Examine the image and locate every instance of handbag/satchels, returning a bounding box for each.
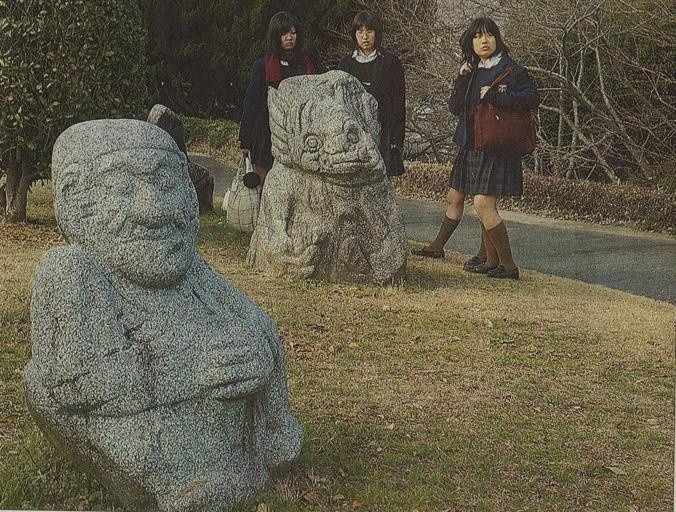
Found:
[224,153,263,233]
[470,101,536,156]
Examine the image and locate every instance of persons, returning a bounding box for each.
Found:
[238,11,312,198]
[337,10,406,179]
[25,117,305,512]
[460,17,537,278]
[410,30,491,272]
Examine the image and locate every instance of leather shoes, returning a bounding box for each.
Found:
[463,254,522,280]
[410,244,445,258]
